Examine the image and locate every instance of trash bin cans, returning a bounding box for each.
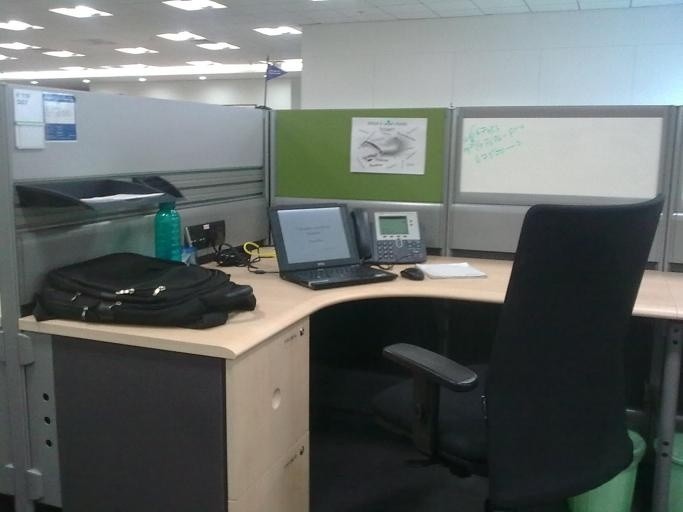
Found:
[566,428,683,512]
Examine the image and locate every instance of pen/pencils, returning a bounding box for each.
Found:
[184,226,193,248]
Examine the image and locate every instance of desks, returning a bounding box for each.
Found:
[18,248,683,512]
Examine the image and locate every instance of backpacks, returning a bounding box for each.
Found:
[32,253,256,329]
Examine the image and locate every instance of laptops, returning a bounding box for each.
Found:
[267,204,397,290]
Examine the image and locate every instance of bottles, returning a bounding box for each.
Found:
[155,200,181,262]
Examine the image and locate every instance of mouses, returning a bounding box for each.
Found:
[400,268,424,280]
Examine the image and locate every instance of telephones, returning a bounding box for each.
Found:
[350,208,427,264]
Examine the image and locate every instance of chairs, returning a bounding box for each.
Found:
[372,194,666,511]
